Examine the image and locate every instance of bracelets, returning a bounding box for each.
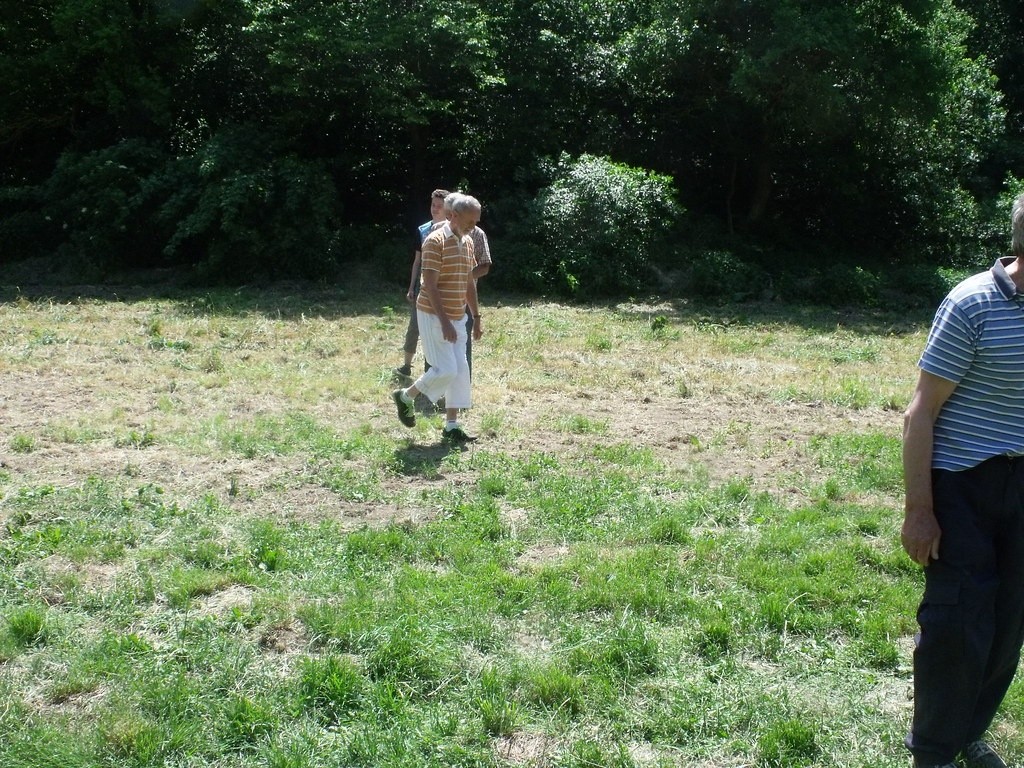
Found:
[472,314,482,319]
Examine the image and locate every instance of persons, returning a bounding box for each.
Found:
[902,189,1024,768]
[392,189,451,377]
[392,196,484,442]
[425,192,492,381]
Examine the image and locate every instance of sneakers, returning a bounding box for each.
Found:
[442,427,479,444]
[392,388,416,427]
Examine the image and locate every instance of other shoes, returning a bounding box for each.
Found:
[395,364,413,376]
[963,737,1008,768]
[910,753,960,768]
